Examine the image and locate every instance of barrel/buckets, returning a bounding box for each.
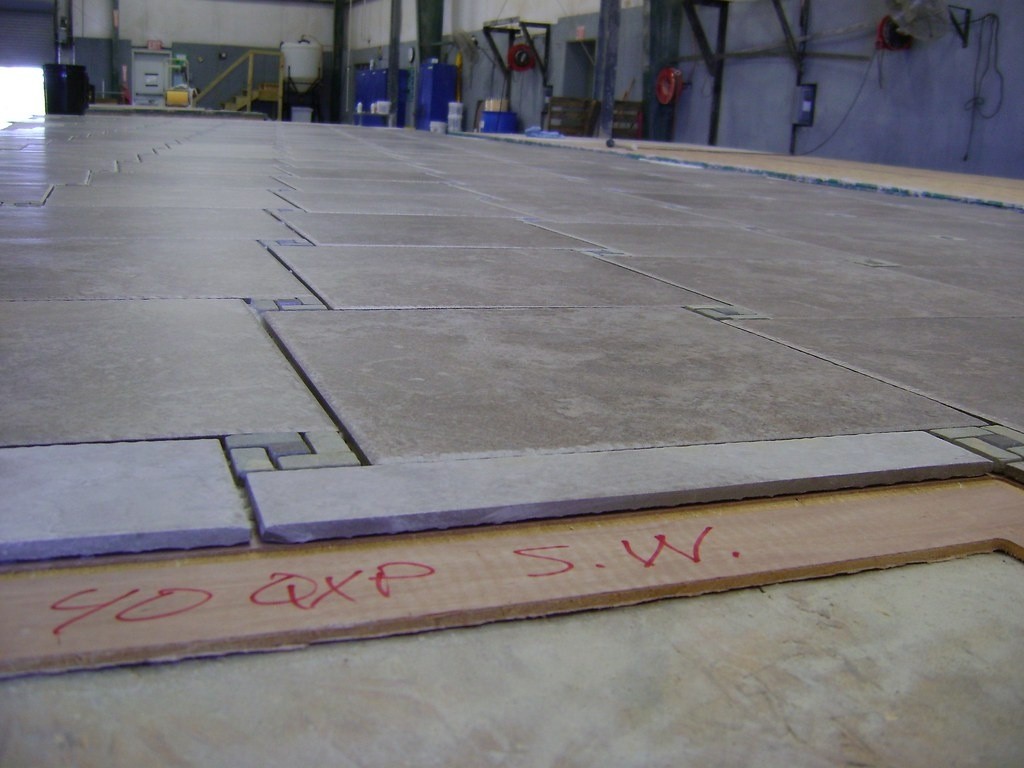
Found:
[429,121,448,134]
[448,103,464,133]
[478,110,519,135]
[376,101,392,114]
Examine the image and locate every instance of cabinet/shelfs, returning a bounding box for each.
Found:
[130,47,172,106]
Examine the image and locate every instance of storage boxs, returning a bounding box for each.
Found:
[485,98,510,111]
[480,110,517,134]
[43,63,89,116]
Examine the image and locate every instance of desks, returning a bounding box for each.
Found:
[415,63,457,132]
[356,69,409,127]
[353,111,392,127]
